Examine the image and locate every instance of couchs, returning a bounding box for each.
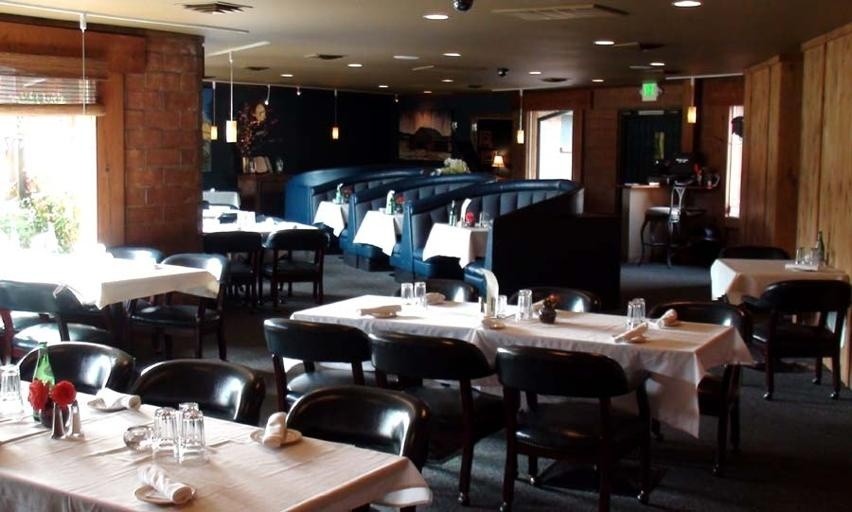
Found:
[285,166,586,299]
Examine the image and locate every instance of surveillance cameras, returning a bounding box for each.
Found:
[451,0,474,14]
[496,67,509,78]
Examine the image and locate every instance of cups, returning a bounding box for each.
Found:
[2,361,25,417]
[794,245,822,266]
[396,280,655,330]
[122,401,207,459]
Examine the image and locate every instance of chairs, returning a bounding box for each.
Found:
[329,280,755,512]
[641,180,722,268]
[0,203,329,512]
[712,245,851,402]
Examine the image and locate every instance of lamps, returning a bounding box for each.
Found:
[491,155,506,175]
[224,47,241,145]
[330,86,341,141]
[515,88,526,145]
[685,75,701,127]
[207,78,223,143]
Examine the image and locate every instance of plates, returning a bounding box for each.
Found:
[135,483,196,503]
[249,429,302,448]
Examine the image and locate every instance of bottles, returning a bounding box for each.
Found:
[334,186,494,227]
[813,230,825,263]
[50,400,87,440]
[33,339,53,424]
